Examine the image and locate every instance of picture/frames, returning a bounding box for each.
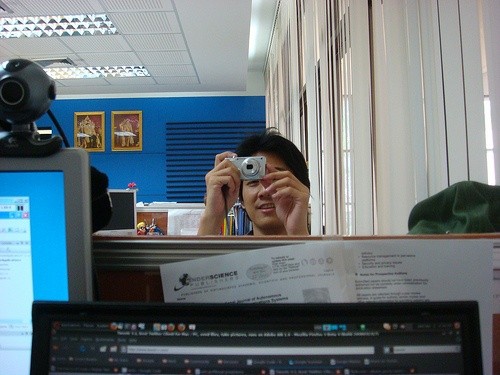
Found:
[110,111,142,152]
[73,111,105,152]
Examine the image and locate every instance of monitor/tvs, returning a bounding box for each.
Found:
[31,299,484,375]
[94,190,136,237]
[0,148,95,375]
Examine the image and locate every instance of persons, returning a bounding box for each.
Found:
[197,127,310,237]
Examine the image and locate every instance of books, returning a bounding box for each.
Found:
[218,206,250,236]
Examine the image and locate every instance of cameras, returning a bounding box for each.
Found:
[223,155,268,180]
